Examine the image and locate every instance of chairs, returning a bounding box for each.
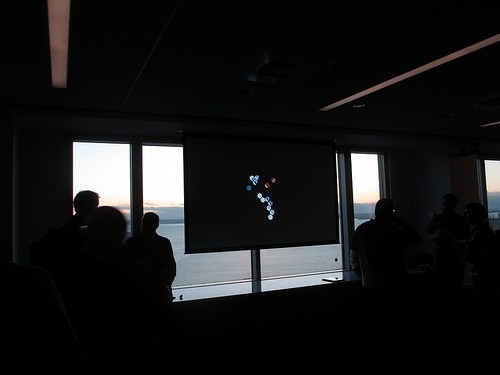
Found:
[0,261,95,375]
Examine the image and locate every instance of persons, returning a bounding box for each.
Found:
[427,194,500,326]
[63,190,176,305]
[350,198,421,299]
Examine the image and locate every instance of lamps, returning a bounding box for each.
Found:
[47,0,71,89]
[318,32,500,112]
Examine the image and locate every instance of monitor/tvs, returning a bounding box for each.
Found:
[182,131,339,254]
